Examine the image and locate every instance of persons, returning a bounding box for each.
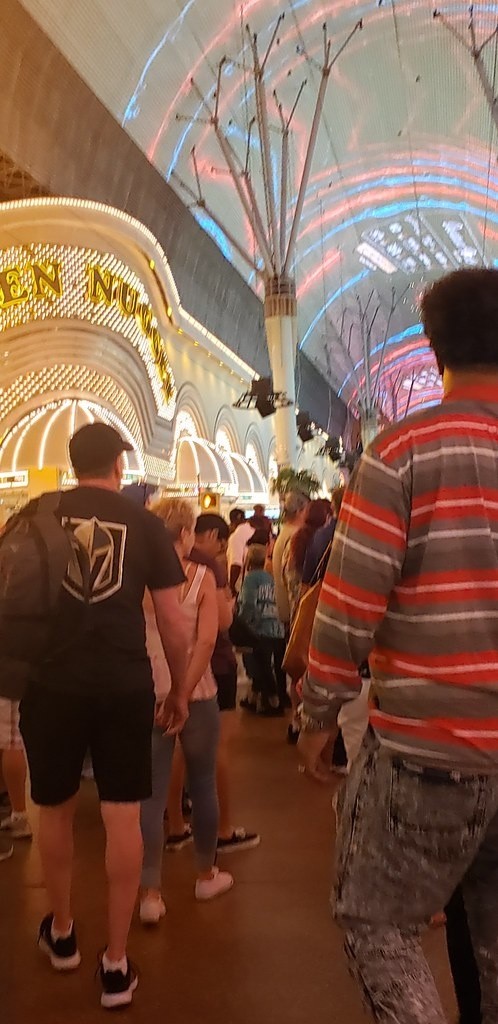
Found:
[0,485,373,925]
[0,422,189,1004]
[297,271,498,1023]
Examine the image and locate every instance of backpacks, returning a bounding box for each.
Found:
[0,489,91,700]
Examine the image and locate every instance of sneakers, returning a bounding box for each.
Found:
[94,944,139,1008]
[37,911,81,970]
[166,822,193,851]
[216,825,260,854]
[140,895,165,925]
[195,865,234,900]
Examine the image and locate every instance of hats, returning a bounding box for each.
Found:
[122,482,160,507]
[70,423,134,471]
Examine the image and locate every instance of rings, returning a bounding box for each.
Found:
[298,765,306,773]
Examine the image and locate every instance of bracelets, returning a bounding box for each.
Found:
[297,703,323,731]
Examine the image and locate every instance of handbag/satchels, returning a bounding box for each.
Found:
[228,617,259,648]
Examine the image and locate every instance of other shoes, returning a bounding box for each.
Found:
[0,835,13,861]
[259,704,284,717]
[0,817,32,840]
[240,698,256,711]
[280,690,292,708]
